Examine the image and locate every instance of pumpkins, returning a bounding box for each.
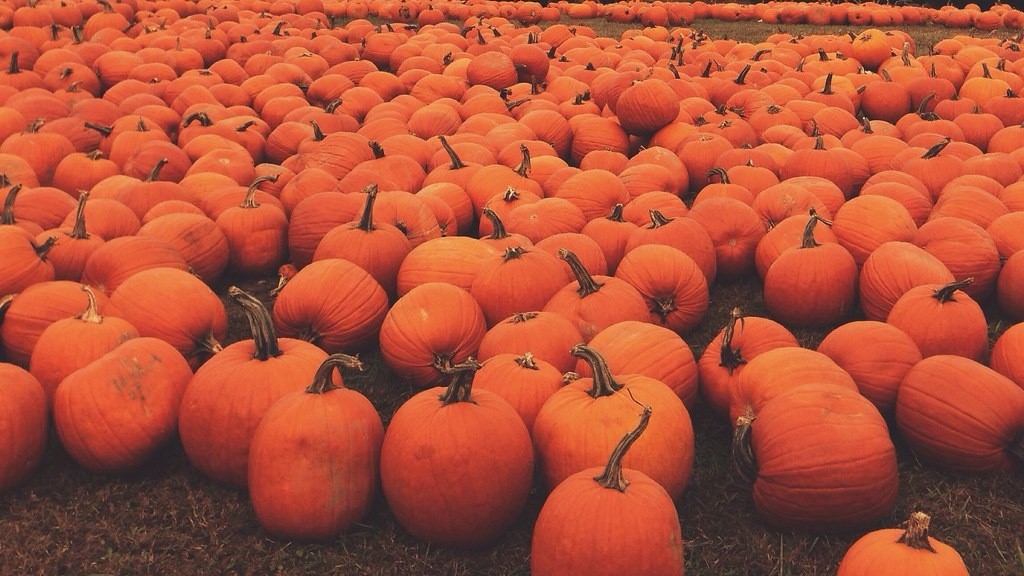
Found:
[0,0,1024,576]
